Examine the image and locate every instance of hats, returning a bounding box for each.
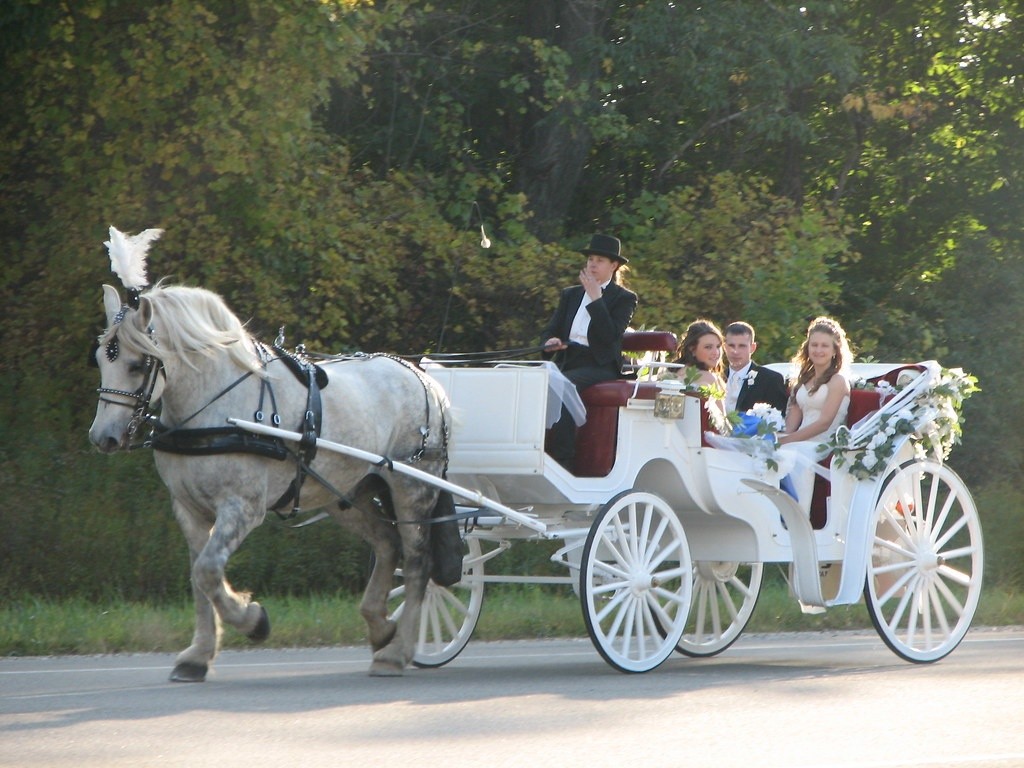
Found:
[580,234,629,265]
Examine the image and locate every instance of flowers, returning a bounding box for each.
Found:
[835,359,982,481]
[748,404,785,431]
[623,322,679,384]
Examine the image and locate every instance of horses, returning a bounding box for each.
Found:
[88,226,465,682]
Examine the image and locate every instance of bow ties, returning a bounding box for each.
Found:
[601,287,604,294]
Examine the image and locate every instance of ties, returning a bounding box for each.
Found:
[729,373,739,410]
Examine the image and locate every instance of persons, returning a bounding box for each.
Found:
[539,234,639,469]
[666,316,856,522]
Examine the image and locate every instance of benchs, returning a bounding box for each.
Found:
[544,381,663,476]
[620,332,731,448]
[782,388,904,481]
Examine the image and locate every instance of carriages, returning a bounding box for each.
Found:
[88,227,987,680]
